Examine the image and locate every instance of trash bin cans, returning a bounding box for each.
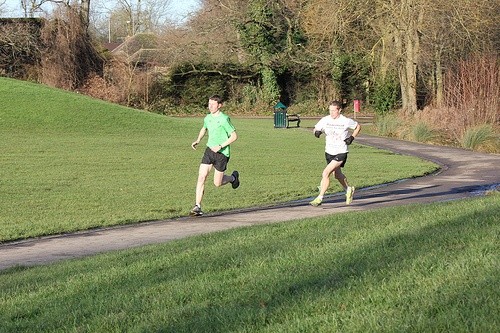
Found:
[273,102,287,129]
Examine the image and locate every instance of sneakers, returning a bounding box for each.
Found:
[230,170,239,188]
[345,186,355,205]
[309,197,323,207]
[190,204,203,215]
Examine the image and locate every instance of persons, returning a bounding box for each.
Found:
[309,101,361,207]
[190,95,240,216]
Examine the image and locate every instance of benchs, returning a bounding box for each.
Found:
[272,111,301,128]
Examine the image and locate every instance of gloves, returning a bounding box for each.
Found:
[315,130,323,138]
[344,135,354,145]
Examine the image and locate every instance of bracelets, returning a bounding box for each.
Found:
[219,144,222,149]
